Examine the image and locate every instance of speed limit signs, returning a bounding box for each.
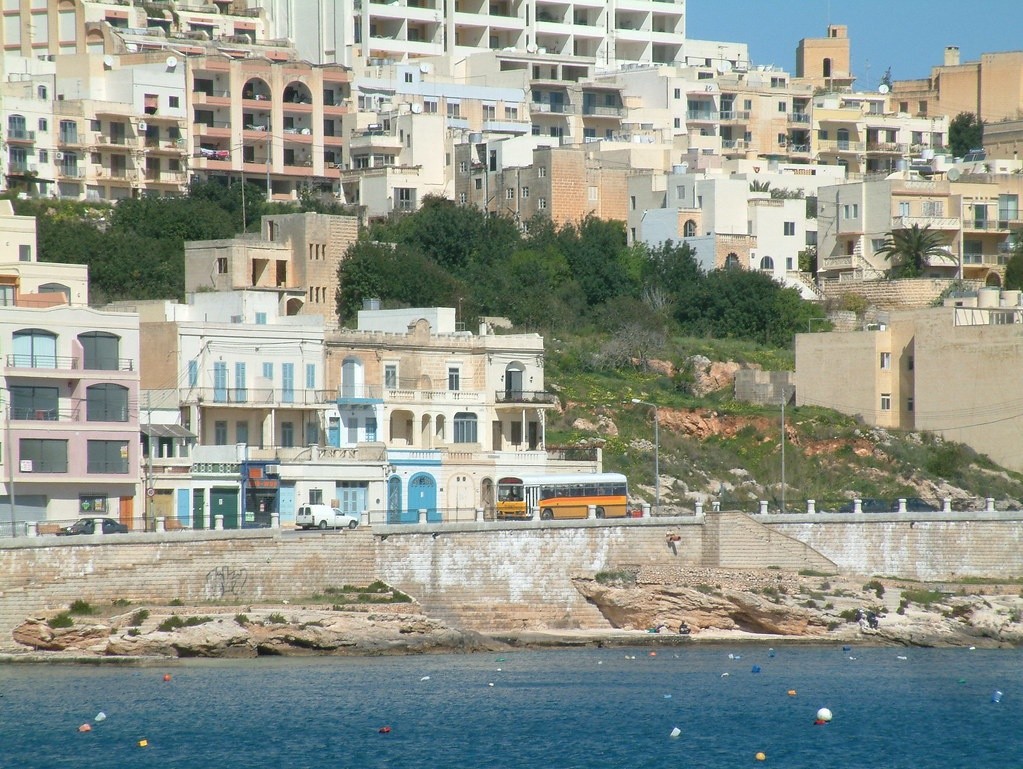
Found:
[146,488,155,496]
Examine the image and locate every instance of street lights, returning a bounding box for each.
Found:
[632,398,659,515]
[781,388,787,512]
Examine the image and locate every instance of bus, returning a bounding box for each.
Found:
[486,472,628,520]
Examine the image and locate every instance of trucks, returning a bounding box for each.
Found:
[296,504,359,529]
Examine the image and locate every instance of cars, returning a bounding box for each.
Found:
[838,497,889,514]
[57,517,127,534]
[891,497,939,513]
